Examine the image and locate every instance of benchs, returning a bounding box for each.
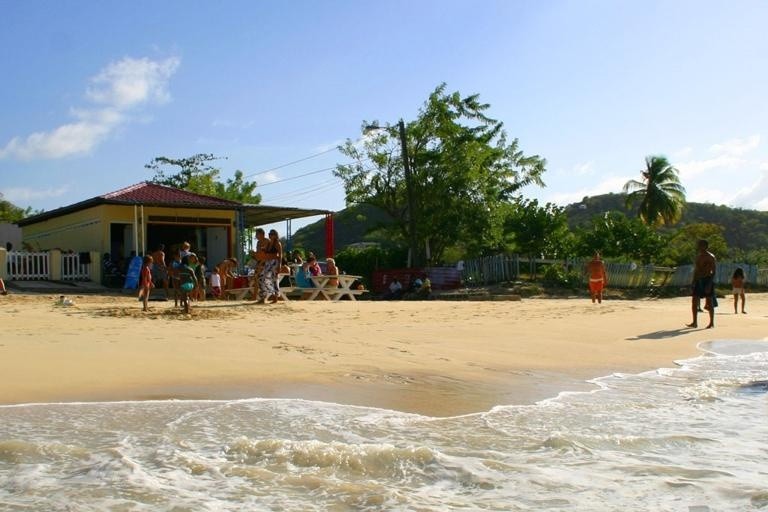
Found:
[224,285,371,300]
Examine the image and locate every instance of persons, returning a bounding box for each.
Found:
[102,228,347,314]
[687,239,716,328]
[403,275,423,299]
[731,268,747,314]
[584,252,607,304]
[416,273,432,300]
[382,277,403,300]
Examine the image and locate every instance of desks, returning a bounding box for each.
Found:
[306,274,363,300]
[237,272,290,300]
